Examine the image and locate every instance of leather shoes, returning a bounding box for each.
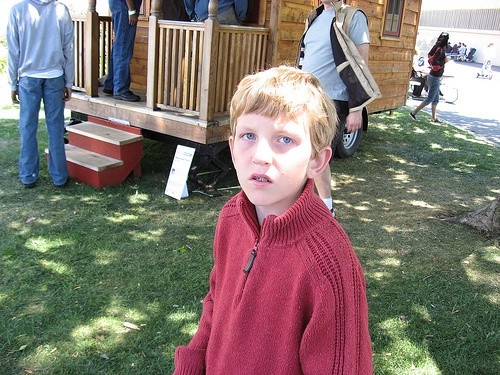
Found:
[103,88,114,94]
[24,180,36,188]
[113,89,141,101]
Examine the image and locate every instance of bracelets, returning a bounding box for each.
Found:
[128,10,137,15]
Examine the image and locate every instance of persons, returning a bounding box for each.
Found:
[172,63,374,375]
[6,0,76,189]
[407,32,452,123]
[446,41,476,63]
[183,0,248,28]
[102,0,142,103]
[292,0,371,218]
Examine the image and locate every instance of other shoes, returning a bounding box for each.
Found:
[431,119,443,124]
[409,112,416,120]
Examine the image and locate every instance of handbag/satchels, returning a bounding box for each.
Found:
[427,47,443,71]
